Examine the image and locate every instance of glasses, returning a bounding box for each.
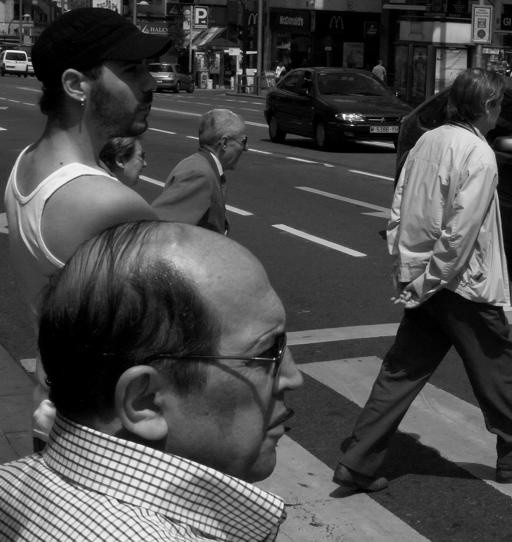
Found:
[230,134,248,147]
[138,331,292,372]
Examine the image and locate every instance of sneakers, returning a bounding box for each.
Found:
[331,462,392,494]
[495,467,511,484]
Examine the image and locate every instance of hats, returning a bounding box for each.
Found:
[30,7,178,83]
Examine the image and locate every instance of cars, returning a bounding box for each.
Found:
[0,49,34,79]
[141,62,194,93]
[264,66,419,152]
[394,75,512,246]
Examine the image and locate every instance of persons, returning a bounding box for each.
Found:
[2,6,164,454]
[0,217,306,542]
[150,105,250,240]
[331,68,510,492]
[99,134,152,188]
[371,59,387,84]
[275,61,286,82]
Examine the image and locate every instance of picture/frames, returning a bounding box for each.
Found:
[471,4,492,45]
